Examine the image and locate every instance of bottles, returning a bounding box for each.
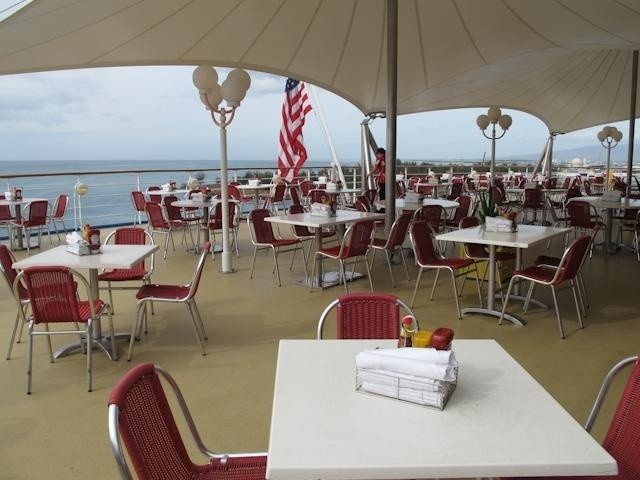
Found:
[169,180,172,190]
[397,316,412,349]
[11,186,16,195]
[322,196,329,205]
[330,200,338,218]
[82,224,91,241]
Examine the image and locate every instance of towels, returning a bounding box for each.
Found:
[354,346,461,409]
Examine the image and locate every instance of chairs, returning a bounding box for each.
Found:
[106,362,269,480]
[496,353,640,480]
[130,172,639,339]
[1,194,211,395]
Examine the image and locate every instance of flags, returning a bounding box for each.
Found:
[273,77,313,184]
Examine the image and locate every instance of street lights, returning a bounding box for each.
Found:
[476,105,512,214]
[192,63,252,274]
[596,126,623,193]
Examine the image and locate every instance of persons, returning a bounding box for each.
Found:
[366,147,385,214]
